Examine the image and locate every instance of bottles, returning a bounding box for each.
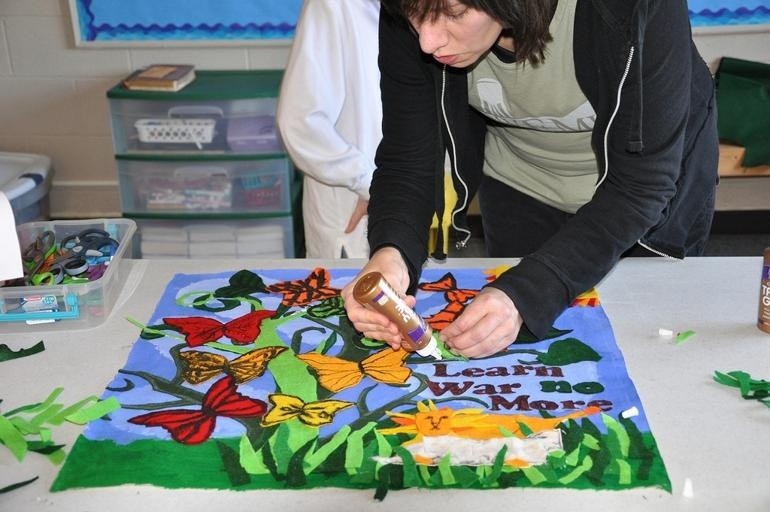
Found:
[757,246,770,332]
[353,273,442,361]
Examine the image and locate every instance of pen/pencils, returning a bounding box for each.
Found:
[147,189,232,210]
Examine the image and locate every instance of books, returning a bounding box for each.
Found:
[138,223,283,260]
[122,63,198,92]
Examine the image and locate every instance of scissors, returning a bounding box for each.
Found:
[3,228,119,306]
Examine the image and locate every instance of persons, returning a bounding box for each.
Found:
[340,0,720,359]
[276,1,383,260]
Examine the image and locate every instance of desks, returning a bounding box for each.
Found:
[1,255,770,512]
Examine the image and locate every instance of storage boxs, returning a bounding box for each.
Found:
[103,72,290,156]
[0,152,53,228]
[111,153,301,213]
[122,212,301,260]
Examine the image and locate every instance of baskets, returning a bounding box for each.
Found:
[134,118,216,144]
